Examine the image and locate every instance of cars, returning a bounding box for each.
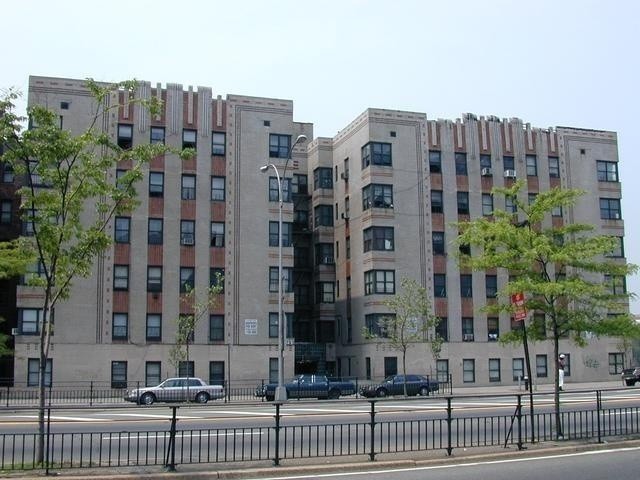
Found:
[125,377,226,403]
[256,374,439,401]
[622,367,640,386]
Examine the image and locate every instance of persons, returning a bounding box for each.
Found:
[557,354,567,390]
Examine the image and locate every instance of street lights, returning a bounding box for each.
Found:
[259,134,308,402]
